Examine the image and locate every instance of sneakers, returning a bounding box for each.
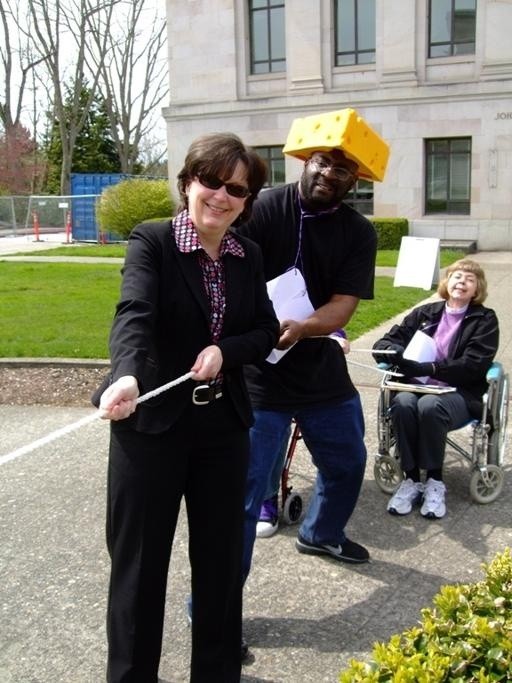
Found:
[256,493,280,536]
[295,531,368,564]
[421,479,446,519]
[387,479,424,515]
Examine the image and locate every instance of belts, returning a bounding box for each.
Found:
[192,385,224,406]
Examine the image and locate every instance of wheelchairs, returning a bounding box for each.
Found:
[372,359,510,503]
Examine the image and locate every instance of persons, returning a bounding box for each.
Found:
[371,259,499,518]
[91,131,281,683]
[256,425,292,537]
[235,149,378,564]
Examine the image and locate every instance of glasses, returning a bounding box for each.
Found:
[196,174,253,198]
[312,158,357,182]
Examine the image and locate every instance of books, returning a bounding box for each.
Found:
[383,380,456,395]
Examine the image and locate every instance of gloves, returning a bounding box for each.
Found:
[385,343,405,364]
[395,358,435,380]
